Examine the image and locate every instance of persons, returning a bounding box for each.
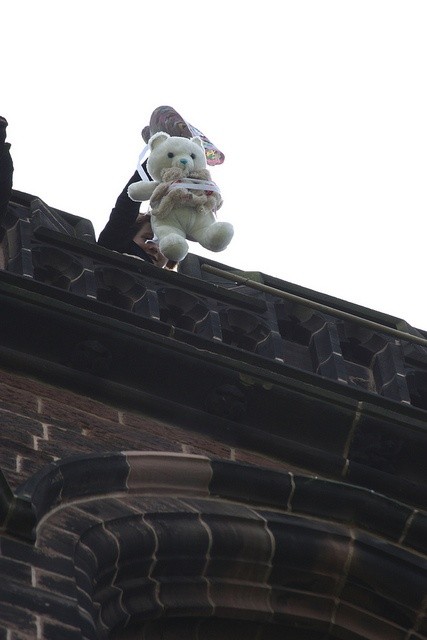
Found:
[97,157,176,269]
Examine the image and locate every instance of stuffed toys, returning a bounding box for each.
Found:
[127,131,234,263]
